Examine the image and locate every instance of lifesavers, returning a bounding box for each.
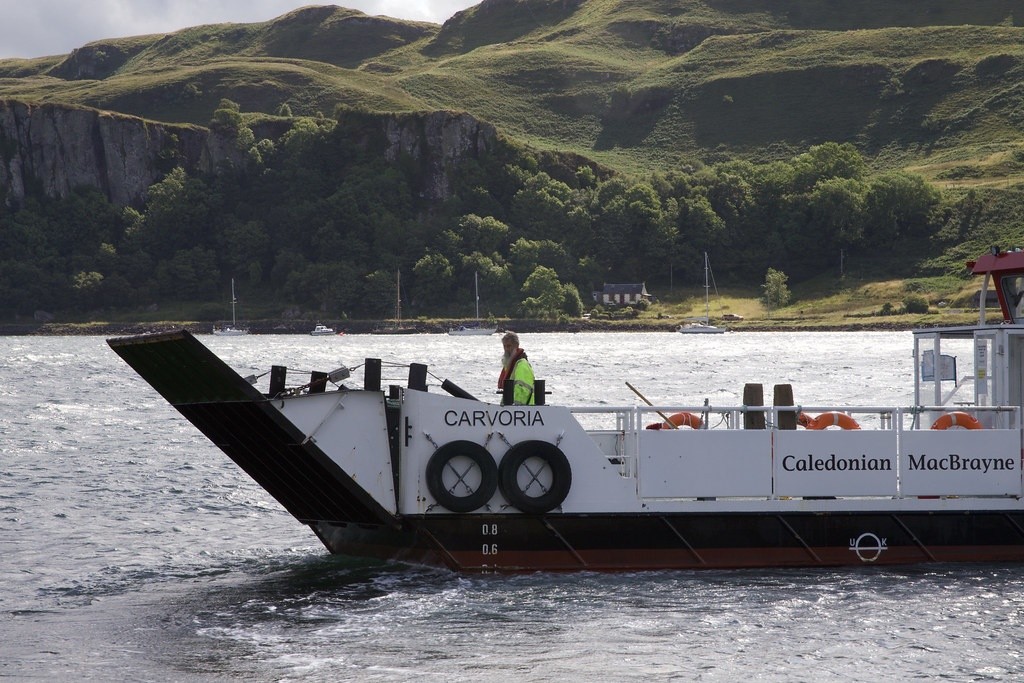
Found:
[425,440,498,512]
[796,412,827,430]
[807,412,861,431]
[498,439,572,513]
[931,412,984,429]
[662,413,705,430]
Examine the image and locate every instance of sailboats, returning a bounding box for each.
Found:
[675,252,726,333]
[449,269,497,335]
[371,266,425,335]
[212,278,251,335]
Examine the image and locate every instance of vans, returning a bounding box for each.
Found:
[582,314,591,320]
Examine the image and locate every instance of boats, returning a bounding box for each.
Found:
[311,325,337,335]
[105,249,1024,576]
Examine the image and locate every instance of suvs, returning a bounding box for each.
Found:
[722,314,743,321]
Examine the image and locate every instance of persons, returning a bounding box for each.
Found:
[498,332,535,406]
